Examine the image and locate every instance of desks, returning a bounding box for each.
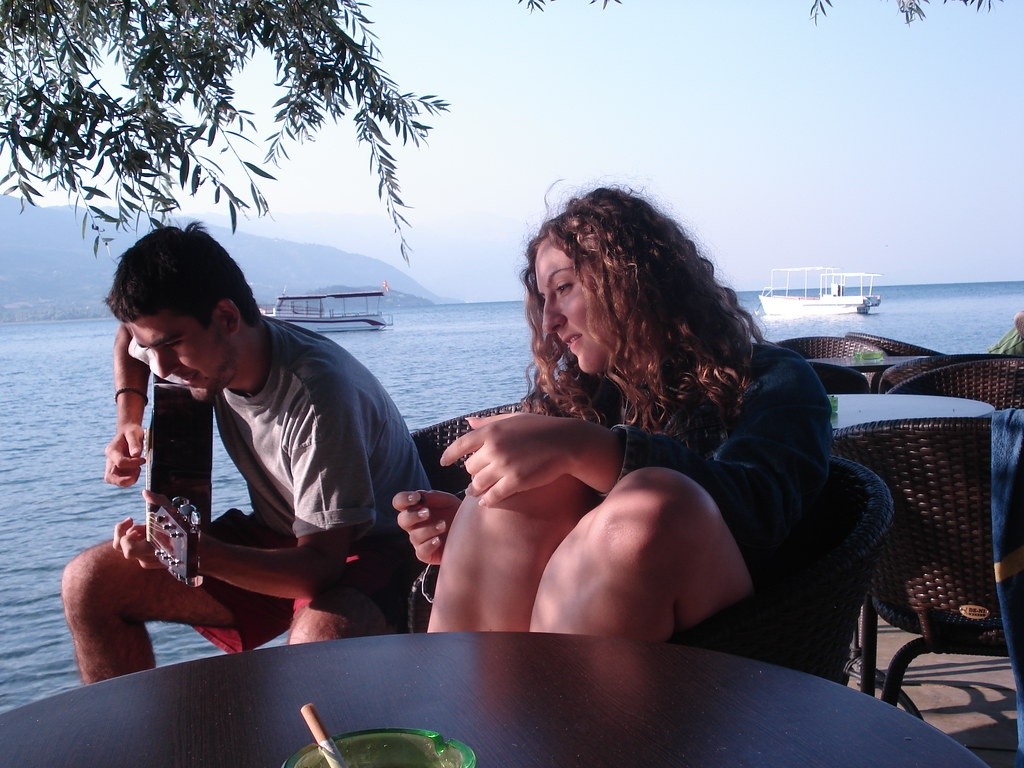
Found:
[819,385,996,696]
[0,620,992,768]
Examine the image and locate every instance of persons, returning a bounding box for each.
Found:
[988,311,1024,356]
[61,188,835,683]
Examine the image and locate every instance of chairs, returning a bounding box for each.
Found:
[386,329,1024,711]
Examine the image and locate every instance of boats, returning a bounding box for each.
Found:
[261,288,394,335]
[758,266,882,317]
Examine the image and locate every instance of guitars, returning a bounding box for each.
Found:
[144,373,214,588]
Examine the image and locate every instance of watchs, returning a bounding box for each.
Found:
[115,387,148,406]
